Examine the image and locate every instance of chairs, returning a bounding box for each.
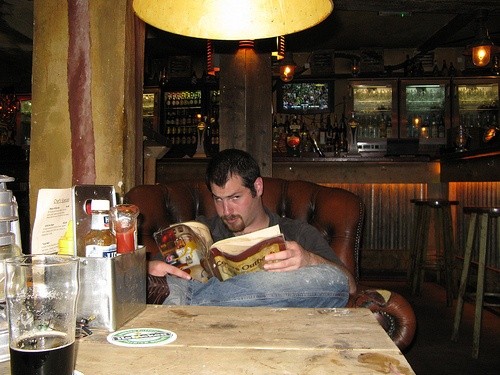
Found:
[122,178,414,352]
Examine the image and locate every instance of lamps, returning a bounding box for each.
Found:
[463,11,500,67]
[239,40,255,49]
[279,52,296,82]
[133,0,334,41]
[278,35,285,56]
[207,39,215,76]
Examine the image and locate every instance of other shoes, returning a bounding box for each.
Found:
[146,272,170,305]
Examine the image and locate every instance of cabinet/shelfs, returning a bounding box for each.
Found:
[143,81,220,157]
[348,76,500,150]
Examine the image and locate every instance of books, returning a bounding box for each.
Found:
[154,222,287,282]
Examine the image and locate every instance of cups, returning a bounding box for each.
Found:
[4,254,80,375]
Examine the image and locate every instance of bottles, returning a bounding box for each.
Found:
[432,60,439,77]
[110,204,139,254]
[269,111,359,158]
[455,113,500,154]
[82,199,117,258]
[143,60,219,158]
[449,62,456,77]
[56,220,75,256]
[403,54,423,77]
[357,113,393,139]
[442,60,448,76]
[405,111,445,140]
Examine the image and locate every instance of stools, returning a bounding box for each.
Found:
[453,207,500,360]
[410,198,458,314]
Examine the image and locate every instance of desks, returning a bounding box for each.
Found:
[0,304,415,375]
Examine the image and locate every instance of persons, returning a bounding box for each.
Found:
[147,149,355,308]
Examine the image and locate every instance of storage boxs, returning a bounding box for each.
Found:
[44,246,146,332]
